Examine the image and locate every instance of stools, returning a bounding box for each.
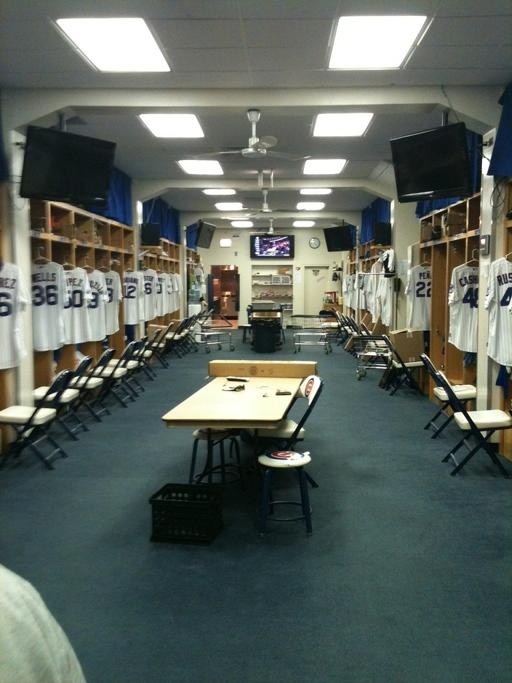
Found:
[258,451,313,539]
[189,427,241,489]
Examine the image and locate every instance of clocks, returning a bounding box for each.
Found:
[309,237,320,248]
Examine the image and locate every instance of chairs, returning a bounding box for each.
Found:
[435,371,511,479]
[0,369,68,470]
[197,308,214,325]
[335,311,348,346]
[381,335,424,396]
[240,375,325,488]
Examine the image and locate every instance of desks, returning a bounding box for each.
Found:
[249,303,285,351]
[161,376,303,522]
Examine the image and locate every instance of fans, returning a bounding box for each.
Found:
[190,108,312,234]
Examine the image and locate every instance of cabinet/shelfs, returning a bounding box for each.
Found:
[343,241,392,336]
[252,265,293,328]
[419,184,512,463]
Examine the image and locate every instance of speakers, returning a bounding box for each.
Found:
[141,223,161,246]
[374,222,391,245]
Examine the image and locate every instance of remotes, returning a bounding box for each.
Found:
[227,376,250,382]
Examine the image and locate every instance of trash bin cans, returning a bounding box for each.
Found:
[256,323,275,353]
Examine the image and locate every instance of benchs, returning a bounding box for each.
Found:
[237,324,303,343]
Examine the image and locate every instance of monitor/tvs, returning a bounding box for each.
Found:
[19,125,117,208]
[323,224,356,252]
[389,123,484,204]
[186,221,216,249]
[250,234,294,259]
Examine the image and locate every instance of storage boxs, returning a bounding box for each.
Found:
[390,328,424,363]
